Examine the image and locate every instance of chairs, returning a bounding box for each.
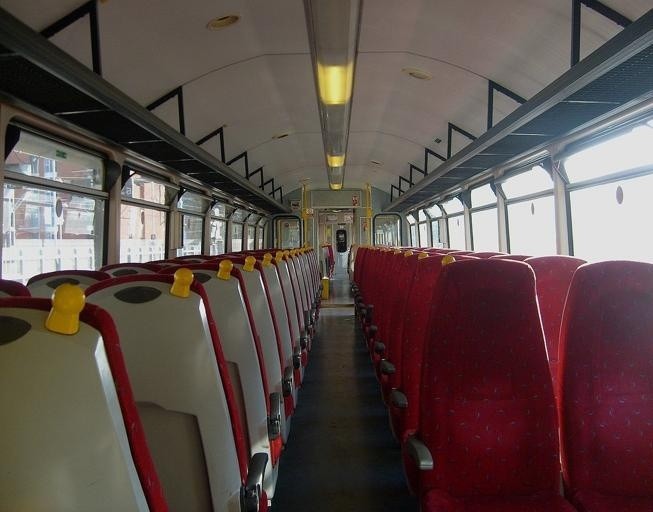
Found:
[351,246,653,512]
[2,246,322,511]
[321,243,335,279]
